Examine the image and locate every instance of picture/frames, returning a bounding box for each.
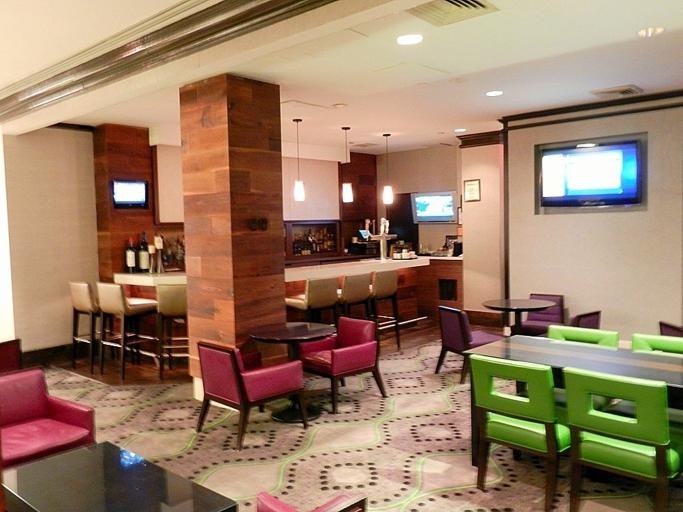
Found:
[462,179,481,202]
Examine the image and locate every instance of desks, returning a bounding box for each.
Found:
[0,440,239,512]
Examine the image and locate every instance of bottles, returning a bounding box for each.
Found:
[124,237,137,273]
[291,227,334,256]
[441,243,447,250]
[136,230,151,273]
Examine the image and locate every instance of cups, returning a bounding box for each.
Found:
[391,249,415,260]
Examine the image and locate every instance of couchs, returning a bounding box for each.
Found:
[0,370,96,472]
[256,487,369,512]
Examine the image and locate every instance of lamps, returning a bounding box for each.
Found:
[292,118,304,200]
[381,133,394,204]
[341,126,354,203]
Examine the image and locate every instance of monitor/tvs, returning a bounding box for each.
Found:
[112,180,148,208]
[410,191,457,224]
[534,131,647,215]
